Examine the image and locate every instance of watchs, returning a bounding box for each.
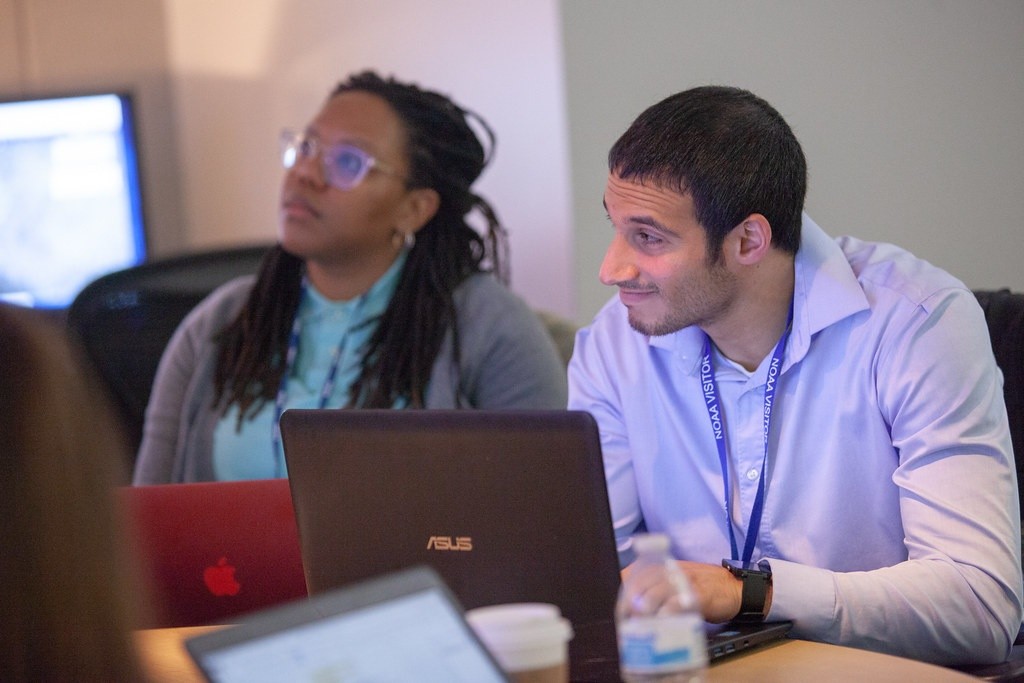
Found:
[722,559,771,626]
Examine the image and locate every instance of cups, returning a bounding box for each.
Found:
[464,605,573,682]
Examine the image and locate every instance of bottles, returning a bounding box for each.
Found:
[614,531,712,683]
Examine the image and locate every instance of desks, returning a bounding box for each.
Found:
[132,619,983,683]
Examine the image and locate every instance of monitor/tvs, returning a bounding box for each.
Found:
[0,94,148,313]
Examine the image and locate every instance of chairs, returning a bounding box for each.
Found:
[0,235,1024,682]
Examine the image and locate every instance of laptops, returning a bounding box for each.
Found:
[113,408,796,683]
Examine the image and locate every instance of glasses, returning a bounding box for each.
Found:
[282,132,412,190]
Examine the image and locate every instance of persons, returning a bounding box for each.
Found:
[564,86,1024,666]
[126,70,567,487]
